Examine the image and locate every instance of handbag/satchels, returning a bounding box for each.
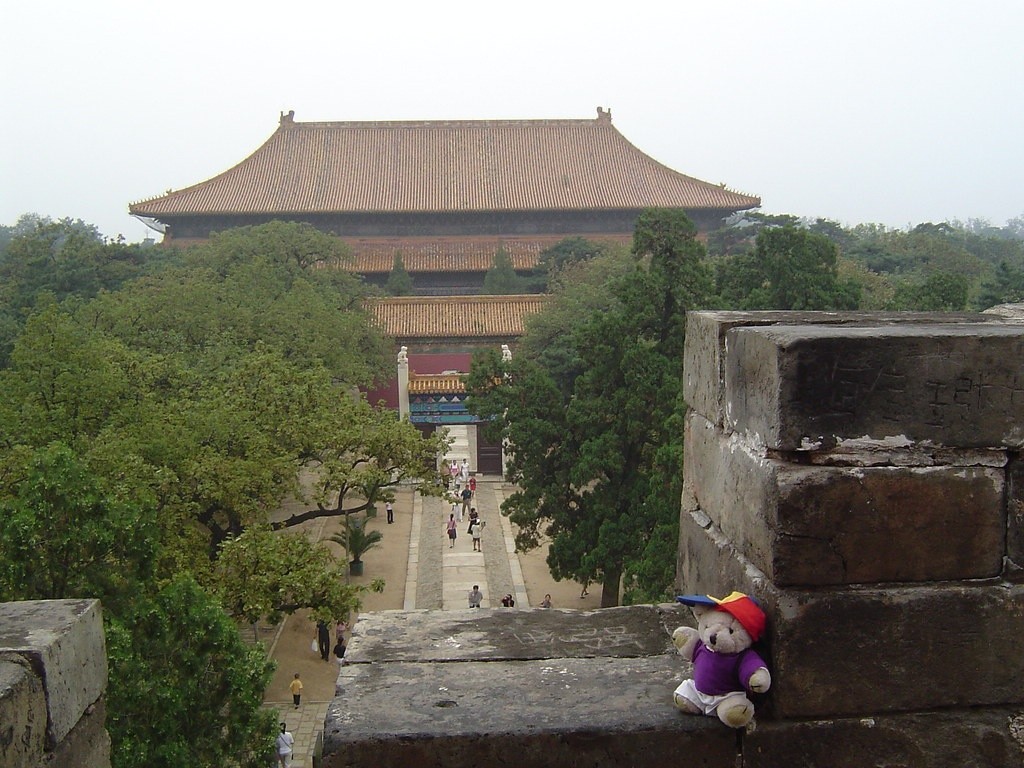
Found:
[312,639,318,652]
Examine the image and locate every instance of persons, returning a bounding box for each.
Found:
[437,456,487,553]
[501,593,514,607]
[538,594,553,608]
[384,493,398,525]
[274,722,295,768]
[310,598,349,667]
[288,673,305,711]
[445,513,457,547]
[467,584,484,608]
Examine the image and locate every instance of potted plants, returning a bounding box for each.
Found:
[331,515,385,576]
[343,487,398,518]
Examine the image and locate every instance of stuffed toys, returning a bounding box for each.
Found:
[669,589,771,729]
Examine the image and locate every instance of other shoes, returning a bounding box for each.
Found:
[473,548,477,551]
[450,545,454,548]
[478,550,481,552]
[295,706,298,709]
[461,520,463,522]
[388,521,394,524]
